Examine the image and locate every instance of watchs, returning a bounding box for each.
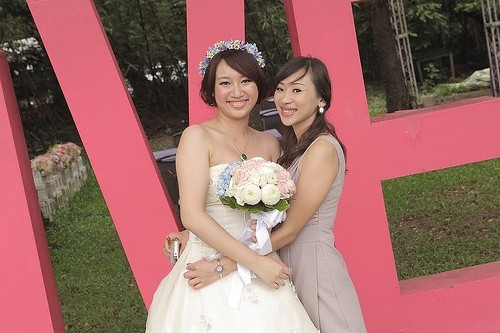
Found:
[215,258,224,279]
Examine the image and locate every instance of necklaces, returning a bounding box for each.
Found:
[216,116,253,161]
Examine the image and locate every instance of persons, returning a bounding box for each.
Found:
[182,56,369,333]
[144,38,322,333]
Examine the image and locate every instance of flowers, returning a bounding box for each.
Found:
[225,157,297,206]
[31,142,82,173]
